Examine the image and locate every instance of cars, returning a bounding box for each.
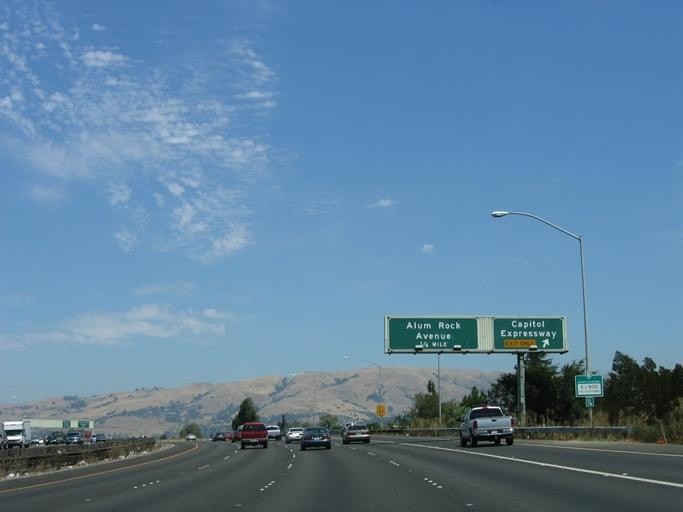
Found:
[210,422,332,450]
[186,434,196,442]
[30,431,112,445]
[341,422,369,444]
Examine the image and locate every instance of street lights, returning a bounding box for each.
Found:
[343,356,382,434]
[492,211,594,422]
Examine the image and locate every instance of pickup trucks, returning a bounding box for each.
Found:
[456,405,514,447]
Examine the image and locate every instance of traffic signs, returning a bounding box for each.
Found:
[384,315,569,355]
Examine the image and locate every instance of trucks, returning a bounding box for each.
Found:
[0,421,31,449]
